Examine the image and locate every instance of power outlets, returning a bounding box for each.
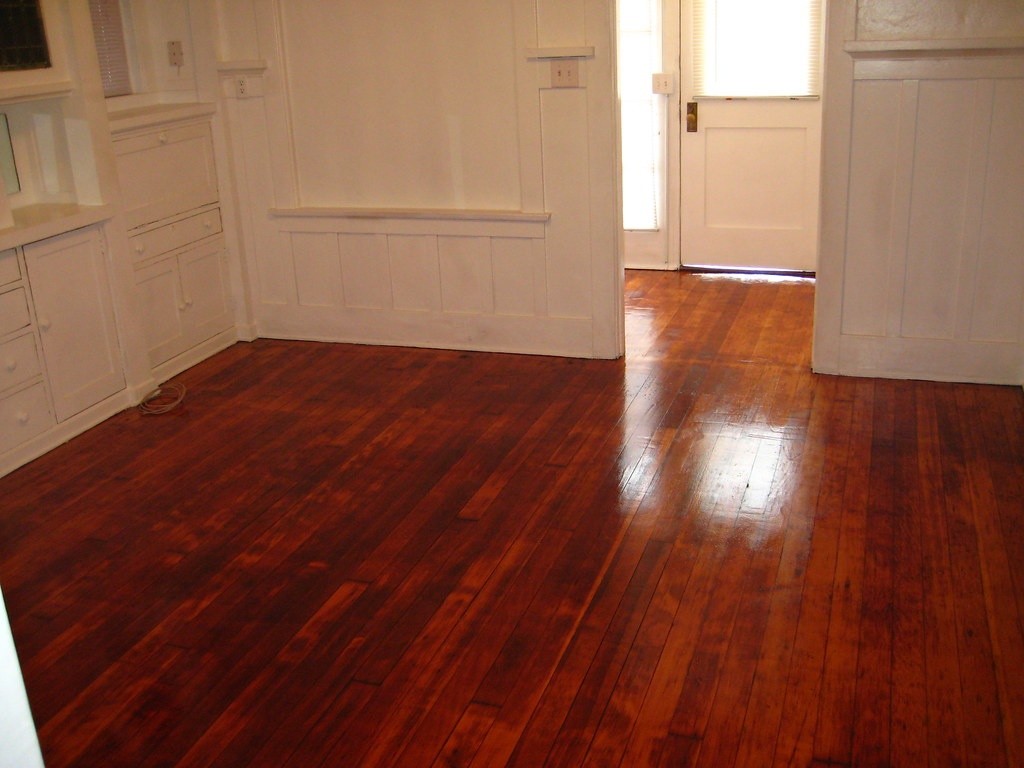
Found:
[237,76,249,98]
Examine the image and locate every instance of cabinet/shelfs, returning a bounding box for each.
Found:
[24,220,127,425]
[102,117,236,372]
[1,248,54,456]
